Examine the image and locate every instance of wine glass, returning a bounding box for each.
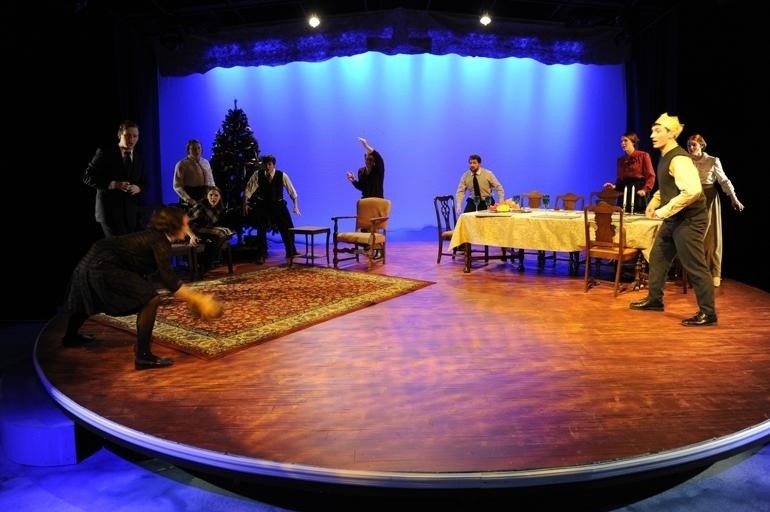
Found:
[542,194,550,216]
[485,196,492,212]
[474,196,480,214]
[513,192,520,206]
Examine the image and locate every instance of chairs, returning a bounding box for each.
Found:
[331,197,391,270]
[554,193,585,211]
[520,192,547,208]
[178,186,232,284]
[589,185,624,205]
[434,195,465,263]
[583,201,641,297]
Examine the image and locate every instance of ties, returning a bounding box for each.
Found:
[267,173,272,182]
[473,174,482,202]
[124,151,131,178]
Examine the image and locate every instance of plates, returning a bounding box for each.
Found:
[491,208,532,213]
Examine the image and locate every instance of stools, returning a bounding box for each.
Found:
[288,227,330,266]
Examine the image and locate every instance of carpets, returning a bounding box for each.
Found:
[87,262,437,362]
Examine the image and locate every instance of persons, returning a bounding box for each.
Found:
[61,202,221,370]
[83,119,152,237]
[686,132,744,290]
[628,111,718,327]
[601,132,656,216]
[187,186,234,275]
[346,138,385,260]
[243,154,301,265]
[454,154,505,215]
[172,138,216,207]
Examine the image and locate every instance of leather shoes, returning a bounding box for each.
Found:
[713,275,720,287]
[134,357,174,371]
[630,296,664,309]
[681,311,718,325]
[62,334,95,346]
[373,250,383,259]
